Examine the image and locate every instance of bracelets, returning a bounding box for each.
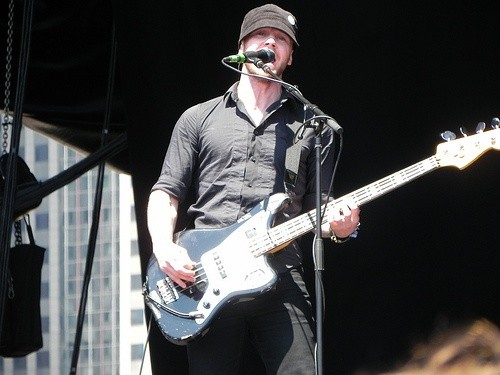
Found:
[329,226,360,244]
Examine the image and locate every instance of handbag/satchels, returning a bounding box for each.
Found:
[0,244,47,357]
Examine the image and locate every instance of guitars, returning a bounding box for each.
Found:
[143,117,500,347]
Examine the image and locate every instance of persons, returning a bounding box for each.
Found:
[147,3,360,375]
[394,320,500,375]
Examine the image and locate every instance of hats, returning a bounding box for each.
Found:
[238,4,301,48]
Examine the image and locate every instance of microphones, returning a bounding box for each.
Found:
[224,48,275,63]
